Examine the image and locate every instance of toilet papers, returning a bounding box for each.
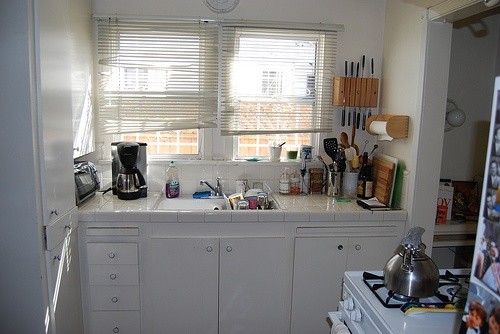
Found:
[369,120,394,142]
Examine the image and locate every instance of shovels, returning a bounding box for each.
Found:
[324,138,338,172]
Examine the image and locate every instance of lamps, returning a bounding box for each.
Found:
[442,97,466,133]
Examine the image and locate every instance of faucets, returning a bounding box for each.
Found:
[200,176,223,199]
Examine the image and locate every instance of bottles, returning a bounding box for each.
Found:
[363,165,373,199]
[356,152,368,198]
[279,168,290,194]
[166,161,179,198]
[290,168,300,194]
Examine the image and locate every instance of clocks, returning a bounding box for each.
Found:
[204,0,240,14]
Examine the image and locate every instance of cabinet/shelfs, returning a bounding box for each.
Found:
[146,235,287,334]
[79,220,146,333]
[289,233,401,334]
[31,0,84,334]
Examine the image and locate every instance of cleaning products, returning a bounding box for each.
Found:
[165,160,179,198]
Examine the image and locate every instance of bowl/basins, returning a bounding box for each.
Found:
[244,188,263,198]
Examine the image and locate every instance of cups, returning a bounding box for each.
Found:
[343,172,359,198]
[287,151,298,159]
[326,170,343,197]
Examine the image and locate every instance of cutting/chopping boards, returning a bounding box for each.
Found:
[371,156,396,206]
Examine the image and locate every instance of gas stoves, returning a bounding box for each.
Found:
[327,268,471,334]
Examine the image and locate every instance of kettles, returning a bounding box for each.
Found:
[383,242,440,298]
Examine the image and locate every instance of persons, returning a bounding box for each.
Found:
[457,115,500,334]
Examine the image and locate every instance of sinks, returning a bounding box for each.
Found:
[155,196,231,220]
